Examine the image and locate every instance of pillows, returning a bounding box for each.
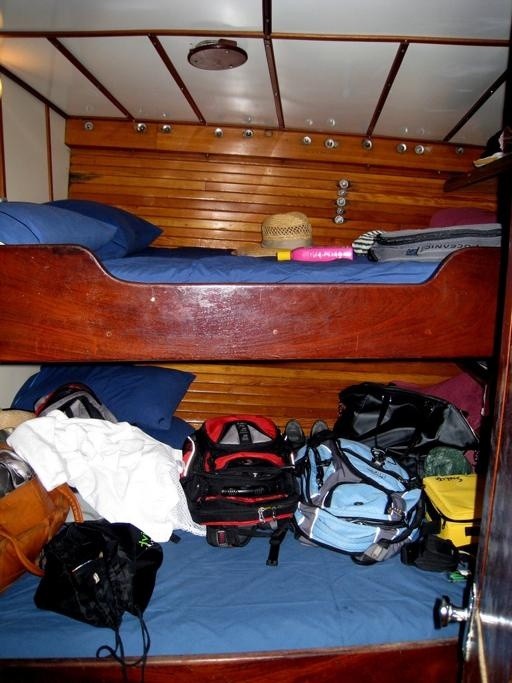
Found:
[2,201,115,258]
[11,365,194,434]
[45,198,161,258]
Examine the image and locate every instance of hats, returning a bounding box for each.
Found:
[232,212,312,260]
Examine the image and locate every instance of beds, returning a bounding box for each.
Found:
[0,248,511,683]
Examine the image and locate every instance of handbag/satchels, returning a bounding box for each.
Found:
[325,370,482,462]
[0,466,82,591]
[395,473,488,572]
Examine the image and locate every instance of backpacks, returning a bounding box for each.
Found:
[284,420,425,566]
[181,415,299,569]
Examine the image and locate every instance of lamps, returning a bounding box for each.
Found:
[187,38,249,72]
